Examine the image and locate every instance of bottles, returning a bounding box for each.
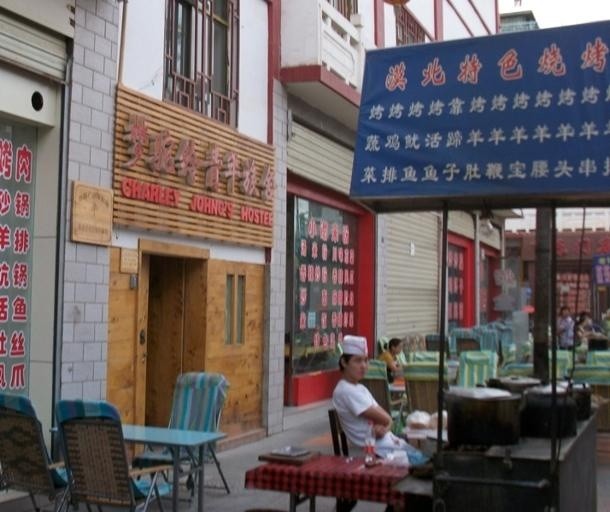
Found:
[363,420,377,466]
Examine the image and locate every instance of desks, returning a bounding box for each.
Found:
[244,452,413,512]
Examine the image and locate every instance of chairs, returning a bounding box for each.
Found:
[1,370,232,512]
[327,408,397,512]
[336,321,610,426]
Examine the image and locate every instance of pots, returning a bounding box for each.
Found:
[441,374,593,447]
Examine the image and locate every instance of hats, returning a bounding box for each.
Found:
[342,335,368,356]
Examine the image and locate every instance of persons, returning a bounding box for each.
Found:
[378,336,405,412]
[556,306,610,353]
[330,333,434,467]
